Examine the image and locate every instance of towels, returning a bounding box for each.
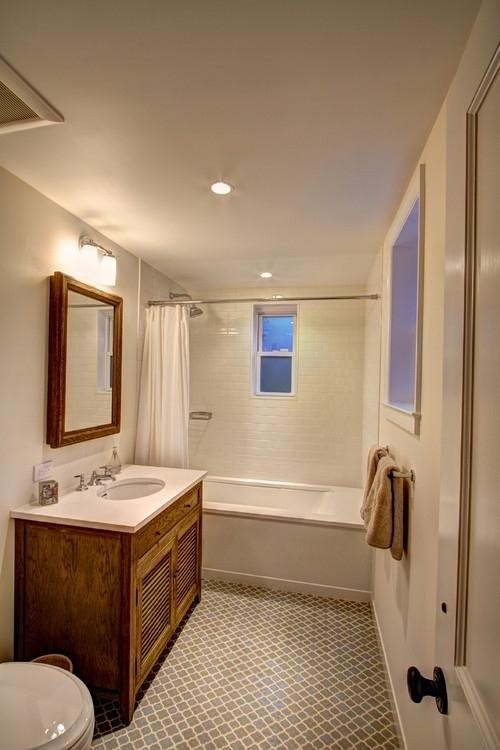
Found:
[360,456,404,560]
[364,446,388,500]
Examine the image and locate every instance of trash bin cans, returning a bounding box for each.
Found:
[29,654,73,673]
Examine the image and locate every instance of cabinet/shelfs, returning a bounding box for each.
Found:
[15,480,203,726]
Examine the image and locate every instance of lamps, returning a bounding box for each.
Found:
[78,234,117,287]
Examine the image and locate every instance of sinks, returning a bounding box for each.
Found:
[95,476,167,502]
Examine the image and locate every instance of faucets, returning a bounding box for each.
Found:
[87,470,107,486]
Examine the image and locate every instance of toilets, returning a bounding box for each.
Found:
[0,659,95,749]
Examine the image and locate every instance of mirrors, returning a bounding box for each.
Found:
[47,271,123,449]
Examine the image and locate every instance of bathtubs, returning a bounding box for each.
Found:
[202,475,375,602]
